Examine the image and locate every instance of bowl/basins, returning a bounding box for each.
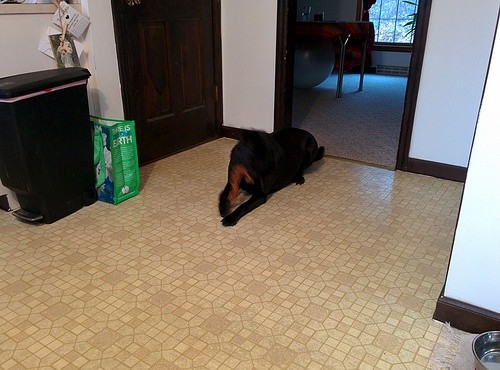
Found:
[472,331,500,370]
[314,14,323,21]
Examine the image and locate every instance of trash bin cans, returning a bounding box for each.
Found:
[0,67,99,226]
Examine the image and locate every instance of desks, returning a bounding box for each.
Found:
[296,20,375,98]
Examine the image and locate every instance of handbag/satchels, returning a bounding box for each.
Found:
[89,115,140,206]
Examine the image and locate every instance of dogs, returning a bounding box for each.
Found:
[218,126,325,227]
